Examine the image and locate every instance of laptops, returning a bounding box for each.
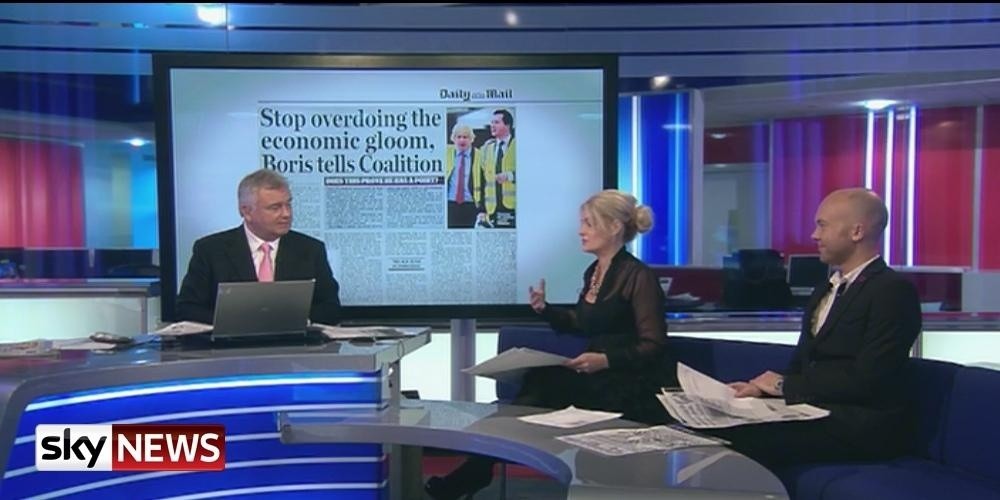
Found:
[177,279,318,348]
[786,253,832,297]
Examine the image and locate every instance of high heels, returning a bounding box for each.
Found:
[426,461,493,499]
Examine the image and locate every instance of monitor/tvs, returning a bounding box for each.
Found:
[150,49,619,323]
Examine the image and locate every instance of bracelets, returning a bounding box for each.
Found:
[774,374,788,394]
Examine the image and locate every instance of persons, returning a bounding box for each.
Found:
[423,187,668,498]
[692,186,922,474]
[446,119,481,228]
[478,109,516,229]
[171,169,345,331]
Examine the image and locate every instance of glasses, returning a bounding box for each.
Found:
[247,198,298,214]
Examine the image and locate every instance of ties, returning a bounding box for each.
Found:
[496,142,505,211]
[457,152,466,205]
[257,243,276,282]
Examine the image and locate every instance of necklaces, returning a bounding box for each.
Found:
[590,260,609,297]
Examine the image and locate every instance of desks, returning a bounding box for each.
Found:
[275,397,791,500]
[0,325,433,500]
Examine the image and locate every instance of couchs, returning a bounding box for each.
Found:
[494,325,1000,500]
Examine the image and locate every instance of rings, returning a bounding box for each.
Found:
[576,367,582,374]
